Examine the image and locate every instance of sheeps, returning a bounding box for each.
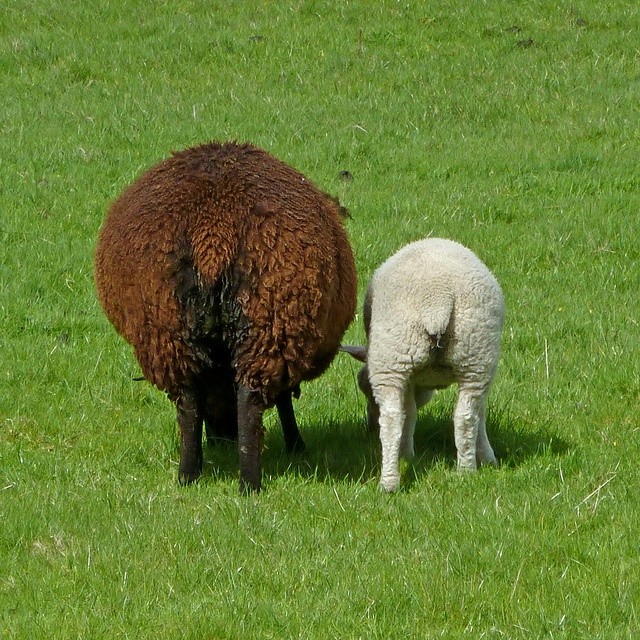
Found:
[337,236,506,494]
[92,137,359,497]
